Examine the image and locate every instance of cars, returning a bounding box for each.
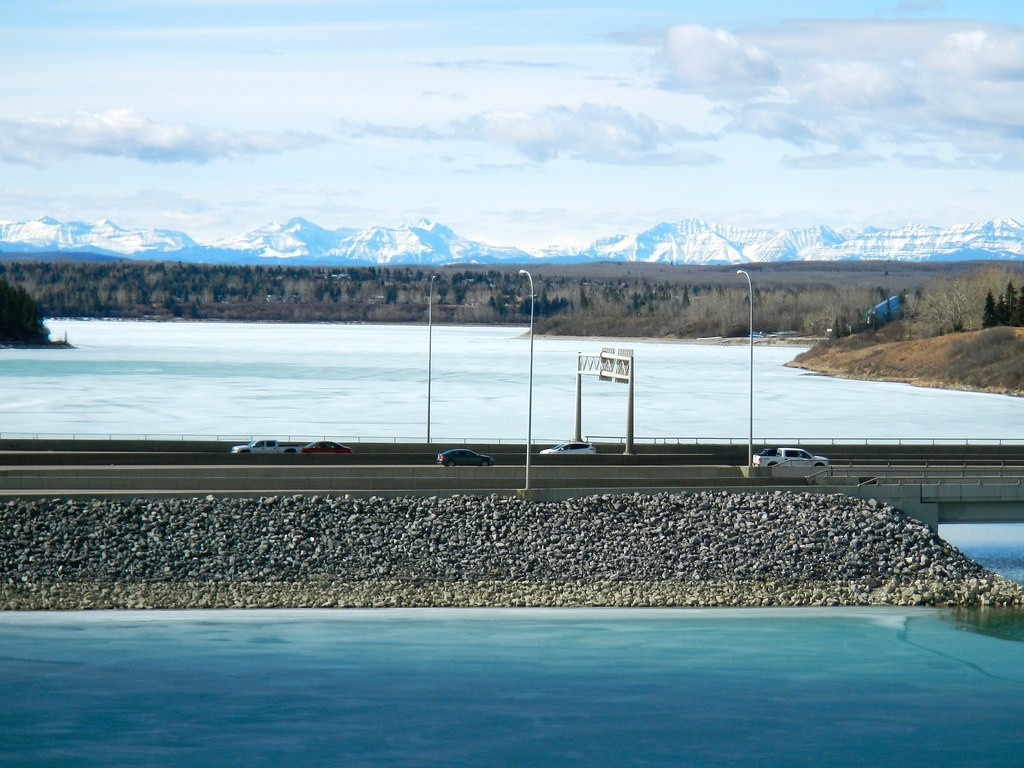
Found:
[302,440,352,453]
[436,448,494,467]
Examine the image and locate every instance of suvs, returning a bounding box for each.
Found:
[540,442,596,454]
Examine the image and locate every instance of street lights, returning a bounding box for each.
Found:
[737,269,753,467]
[427,275,436,444]
[520,269,534,489]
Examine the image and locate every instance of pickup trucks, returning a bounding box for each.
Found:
[231,439,302,453]
[753,447,829,467]
